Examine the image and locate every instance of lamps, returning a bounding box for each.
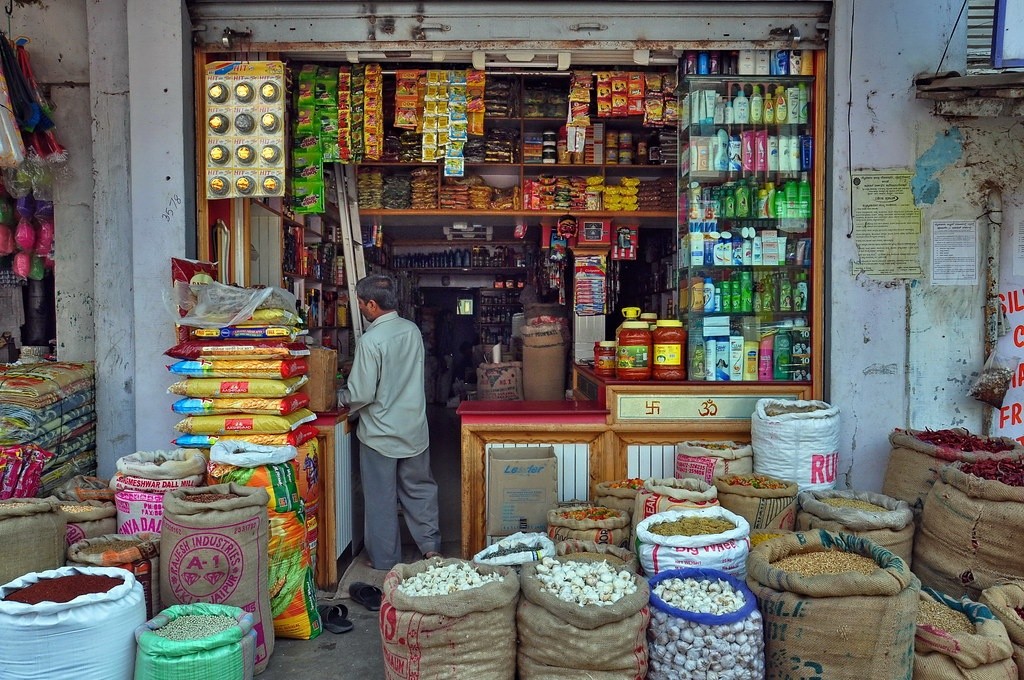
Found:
[770,26,802,44]
[221,25,249,47]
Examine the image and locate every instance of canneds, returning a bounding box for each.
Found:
[615,313,687,382]
[593,340,615,377]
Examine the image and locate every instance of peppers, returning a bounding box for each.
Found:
[905,426,1024,583]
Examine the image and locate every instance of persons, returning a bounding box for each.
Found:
[341,274,441,570]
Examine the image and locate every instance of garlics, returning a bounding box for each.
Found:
[647,578,765,680]
[535,556,638,607]
[398,560,503,596]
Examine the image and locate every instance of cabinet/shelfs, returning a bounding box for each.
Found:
[285,51,826,583]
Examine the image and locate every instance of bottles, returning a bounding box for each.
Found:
[393,245,531,269]
[280,222,391,329]
[593,260,686,381]
[481,274,524,345]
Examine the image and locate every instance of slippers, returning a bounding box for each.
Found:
[317,603,354,634]
[350,582,382,611]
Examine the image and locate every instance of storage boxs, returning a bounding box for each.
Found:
[304,348,339,412]
